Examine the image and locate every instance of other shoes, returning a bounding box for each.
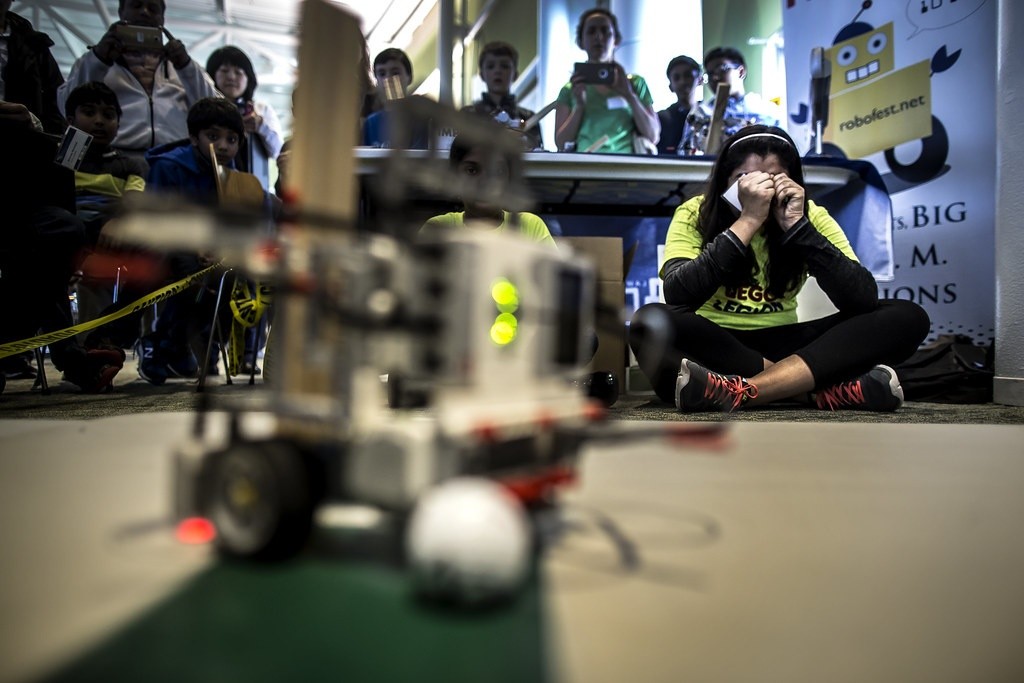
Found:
[242,363,261,374]
[209,366,218,376]
[3,353,38,380]
[61,366,106,394]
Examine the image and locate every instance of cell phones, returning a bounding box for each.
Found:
[575,61,616,85]
[118,25,164,49]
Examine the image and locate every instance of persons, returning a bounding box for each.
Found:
[0,0,375,389]
[655,55,703,154]
[359,46,440,149]
[380,125,620,410]
[554,7,661,285]
[458,40,543,150]
[676,45,785,155]
[628,125,930,414]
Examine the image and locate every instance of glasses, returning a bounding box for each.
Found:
[702,64,737,84]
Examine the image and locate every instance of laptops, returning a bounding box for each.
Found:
[704,83,730,156]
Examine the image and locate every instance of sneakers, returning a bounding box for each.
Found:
[167,343,199,377]
[805,365,904,412]
[133,338,167,384]
[675,358,758,414]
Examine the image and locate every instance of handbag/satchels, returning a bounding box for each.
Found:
[894,341,990,404]
